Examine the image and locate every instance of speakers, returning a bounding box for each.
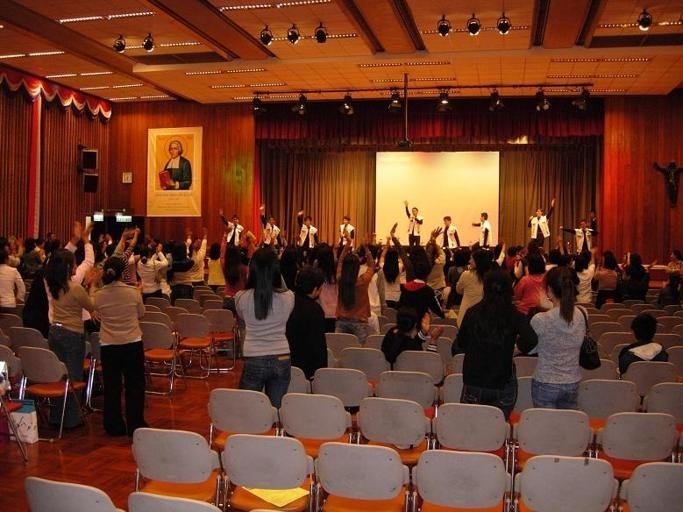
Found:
[83,173,98,193]
[82,149,98,170]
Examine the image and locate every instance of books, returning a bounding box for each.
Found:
[157,170,171,188]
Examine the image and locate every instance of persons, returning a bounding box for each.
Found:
[447,267,540,426]
[1,197,683,389]
[45,245,105,433]
[234,246,296,411]
[161,140,191,190]
[91,255,153,437]
[526,265,590,411]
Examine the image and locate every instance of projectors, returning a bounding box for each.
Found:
[396,139,413,148]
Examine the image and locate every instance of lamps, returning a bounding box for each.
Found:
[257,22,328,47]
[110,32,155,53]
[248,80,597,119]
[435,11,512,37]
[635,9,652,32]
